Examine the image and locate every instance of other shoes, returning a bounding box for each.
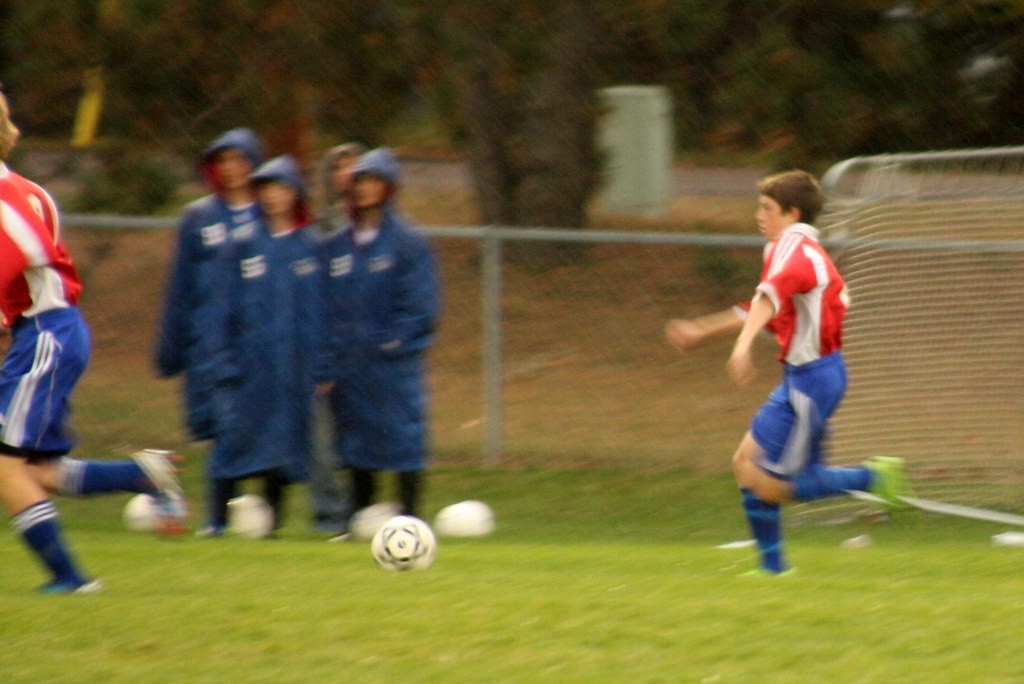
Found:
[326,529,352,543]
[193,522,226,538]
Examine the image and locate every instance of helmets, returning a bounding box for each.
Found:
[195,126,264,172]
[346,145,400,180]
[247,154,301,192]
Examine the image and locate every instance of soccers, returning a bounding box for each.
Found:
[369,512,437,571]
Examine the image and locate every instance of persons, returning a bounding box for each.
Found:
[0,92,181,593]
[663,169,914,574]
[149,126,444,539]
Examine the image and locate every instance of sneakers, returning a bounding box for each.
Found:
[734,566,801,580]
[130,449,185,517]
[864,455,900,506]
[33,576,100,593]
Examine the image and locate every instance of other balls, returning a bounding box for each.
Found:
[227,495,274,537]
[122,493,161,533]
[348,502,401,543]
[435,497,495,538]
